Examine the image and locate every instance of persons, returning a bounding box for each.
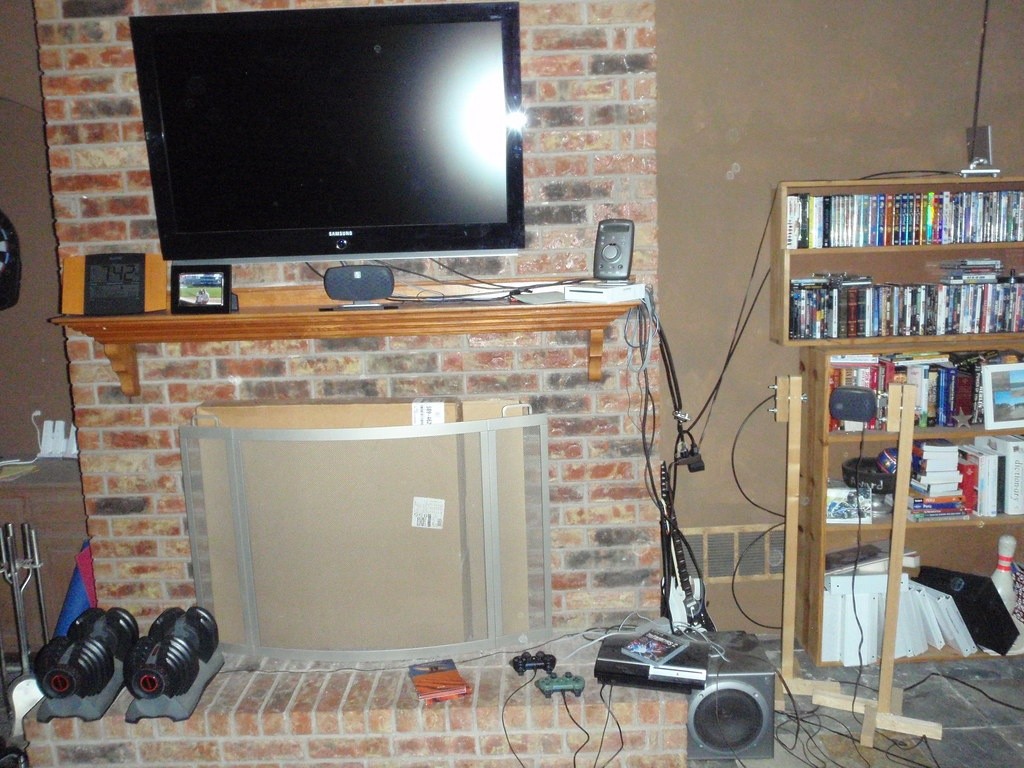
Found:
[196,289,209,305]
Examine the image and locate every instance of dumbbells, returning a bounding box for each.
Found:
[122,605,219,700]
[33,607,140,700]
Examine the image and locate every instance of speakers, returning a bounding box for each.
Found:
[686,631,777,759]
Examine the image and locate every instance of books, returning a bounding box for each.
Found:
[825,353,1024,525]
[790,259,1024,338]
[786,190,1024,249]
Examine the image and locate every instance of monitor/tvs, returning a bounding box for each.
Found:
[129,1,527,265]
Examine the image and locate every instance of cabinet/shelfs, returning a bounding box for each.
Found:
[794,339,1024,667]
[769,176,1024,347]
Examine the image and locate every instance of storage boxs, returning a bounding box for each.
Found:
[196,400,530,650]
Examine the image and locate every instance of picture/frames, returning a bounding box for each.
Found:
[171,264,232,315]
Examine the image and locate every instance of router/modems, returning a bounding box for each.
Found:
[563,282,646,304]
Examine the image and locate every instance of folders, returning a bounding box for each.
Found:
[822,573,977,668]
